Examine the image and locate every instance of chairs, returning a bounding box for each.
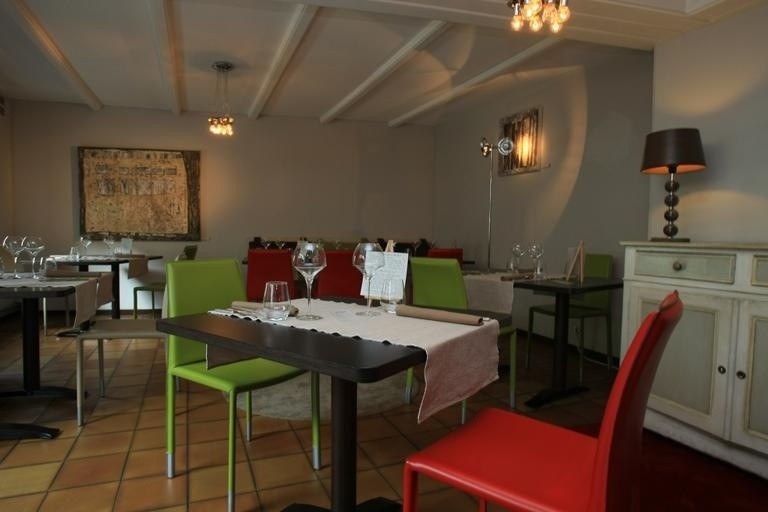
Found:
[75,244,199,426]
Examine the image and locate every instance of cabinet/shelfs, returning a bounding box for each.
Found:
[616,238,768,476]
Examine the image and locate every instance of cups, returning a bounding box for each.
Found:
[264,280,290,320]
[69,235,122,262]
[381,279,405,315]
[257,236,343,252]
[40,256,57,280]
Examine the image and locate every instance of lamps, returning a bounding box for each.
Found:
[639,127,709,241]
[479,137,514,271]
[206,61,236,137]
[505,1,572,35]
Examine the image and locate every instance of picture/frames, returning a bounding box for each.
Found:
[496,106,542,177]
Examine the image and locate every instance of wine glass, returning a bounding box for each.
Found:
[506,241,545,277]
[353,242,385,316]
[290,241,327,323]
[4,235,44,278]
